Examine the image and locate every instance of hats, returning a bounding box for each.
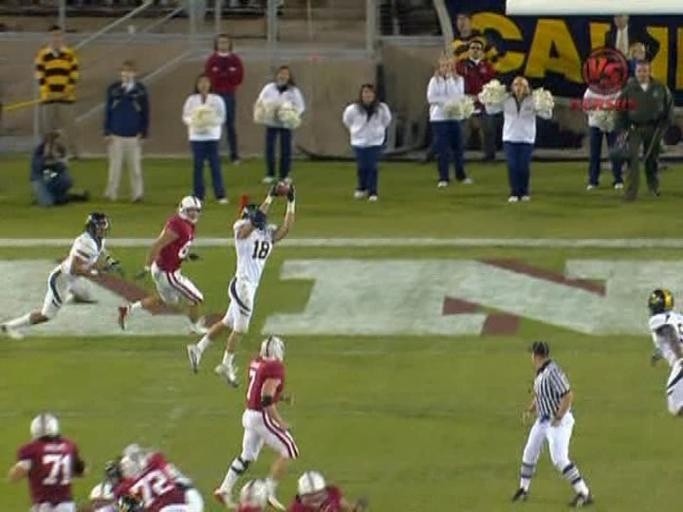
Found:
[527,342,550,355]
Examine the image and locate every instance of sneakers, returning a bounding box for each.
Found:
[514,488,528,501]
[569,488,593,508]
[0,297,240,388]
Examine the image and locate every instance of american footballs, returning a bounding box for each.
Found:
[274,180,290,198]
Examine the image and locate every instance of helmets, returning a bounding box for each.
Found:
[84,212,109,239]
[241,206,266,229]
[30,413,60,439]
[260,335,284,363]
[105,443,327,510]
[178,196,202,225]
[649,289,674,312]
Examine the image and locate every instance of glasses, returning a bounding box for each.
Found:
[471,47,479,51]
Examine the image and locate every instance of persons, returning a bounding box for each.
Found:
[117,196,203,336]
[648,290,682,418]
[214,335,299,510]
[205,34,243,166]
[100,60,150,203]
[181,73,229,205]
[7,411,361,512]
[254,65,306,182]
[0,211,120,340]
[428,14,672,202]
[512,341,596,506]
[342,84,393,201]
[187,179,296,388]
[29,25,89,208]
